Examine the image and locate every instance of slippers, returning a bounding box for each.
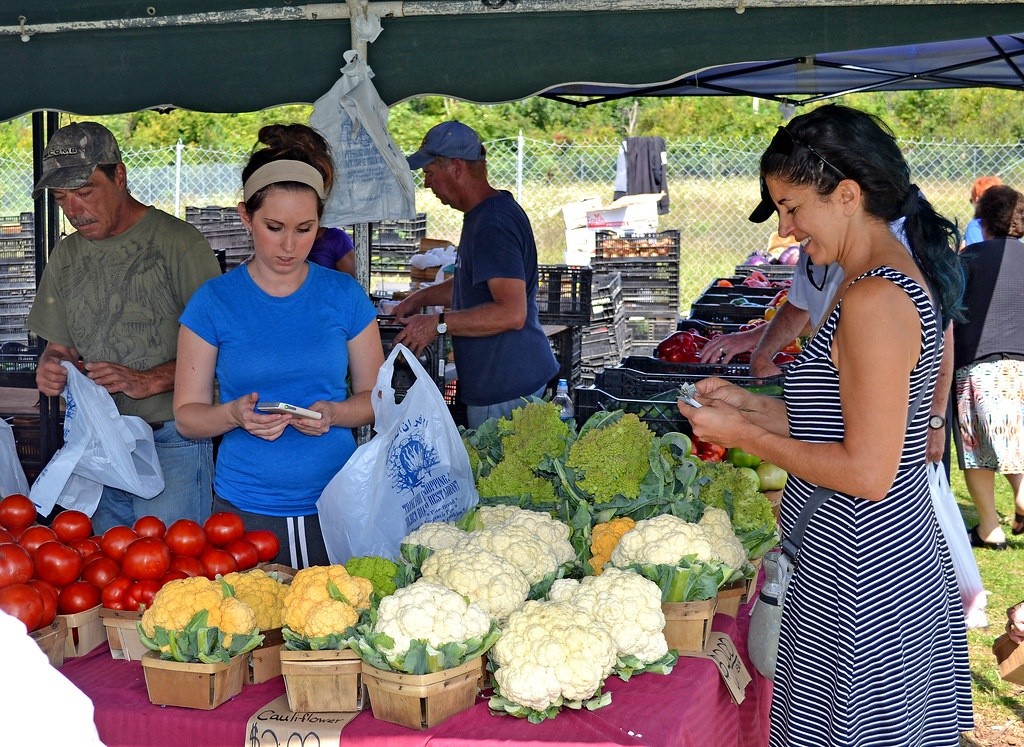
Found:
[1012,512,1024,536]
[967,523,1007,551]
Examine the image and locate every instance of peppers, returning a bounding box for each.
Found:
[657,290,809,362]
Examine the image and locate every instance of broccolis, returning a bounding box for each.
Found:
[465,402,778,561]
[133,502,747,723]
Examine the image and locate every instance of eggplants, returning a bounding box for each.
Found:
[743,246,803,267]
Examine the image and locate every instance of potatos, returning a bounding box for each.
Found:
[598,238,673,258]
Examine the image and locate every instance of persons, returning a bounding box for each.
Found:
[389,117,560,431]
[678,104,989,747]
[23,120,224,533]
[952,176,1024,549]
[174,123,386,569]
[305,224,355,277]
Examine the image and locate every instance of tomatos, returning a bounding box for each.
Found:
[0,493,277,635]
[688,434,788,492]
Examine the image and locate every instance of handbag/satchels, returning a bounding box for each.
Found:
[746,550,793,679]
[926,459,992,631]
[28,360,164,518]
[316,342,478,567]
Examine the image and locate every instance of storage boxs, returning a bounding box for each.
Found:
[0,209,799,522]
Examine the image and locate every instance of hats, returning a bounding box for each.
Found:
[32,121,123,201]
[747,161,778,223]
[406,119,486,169]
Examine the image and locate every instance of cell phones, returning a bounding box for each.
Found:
[257,402,323,420]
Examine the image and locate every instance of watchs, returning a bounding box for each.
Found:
[437,312,447,336]
[928,415,946,430]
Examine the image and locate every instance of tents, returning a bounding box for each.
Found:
[0,0,1024,452]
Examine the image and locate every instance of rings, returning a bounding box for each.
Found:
[717,347,724,354]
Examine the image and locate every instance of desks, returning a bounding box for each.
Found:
[57,550,783,747]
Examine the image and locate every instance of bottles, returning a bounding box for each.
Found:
[551,378,575,423]
